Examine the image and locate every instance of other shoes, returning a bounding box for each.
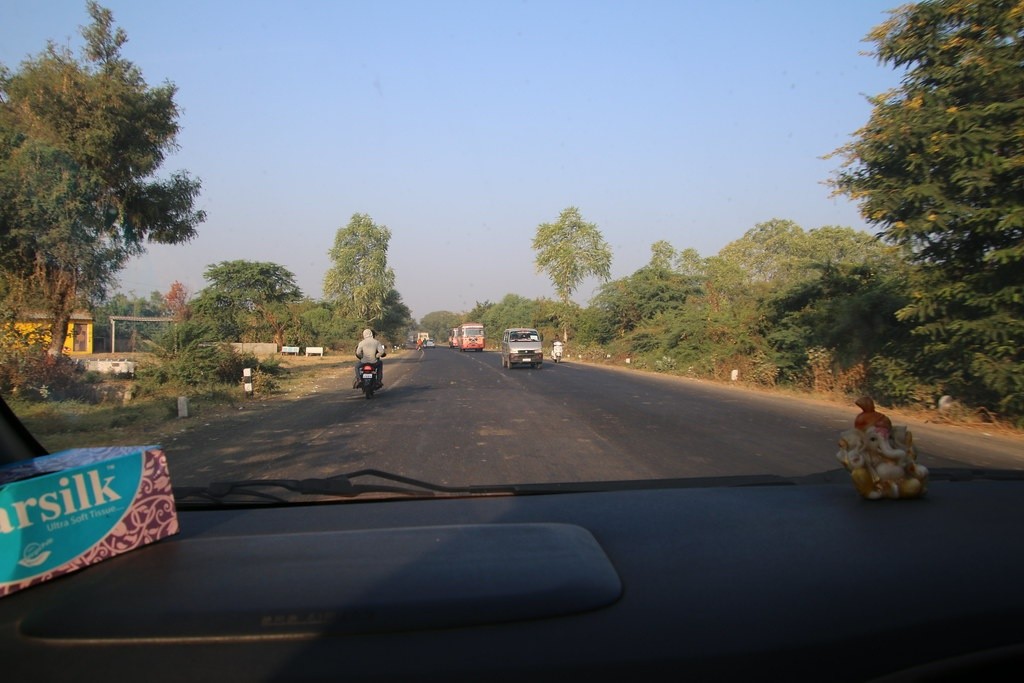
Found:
[354,379,363,387]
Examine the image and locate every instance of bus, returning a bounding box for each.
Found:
[447,328,458,348]
[458,323,485,352]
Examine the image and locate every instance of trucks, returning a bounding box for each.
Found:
[416,332,429,345]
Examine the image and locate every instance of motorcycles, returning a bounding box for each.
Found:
[550,340,565,364]
[354,352,386,399]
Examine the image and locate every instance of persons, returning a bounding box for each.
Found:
[552,335,562,343]
[417,338,422,346]
[423,338,427,349]
[353,329,385,389]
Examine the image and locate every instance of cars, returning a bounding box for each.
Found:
[430,337,434,341]
[427,341,435,347]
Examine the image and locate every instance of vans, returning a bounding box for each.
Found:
[501,326,544,370]
